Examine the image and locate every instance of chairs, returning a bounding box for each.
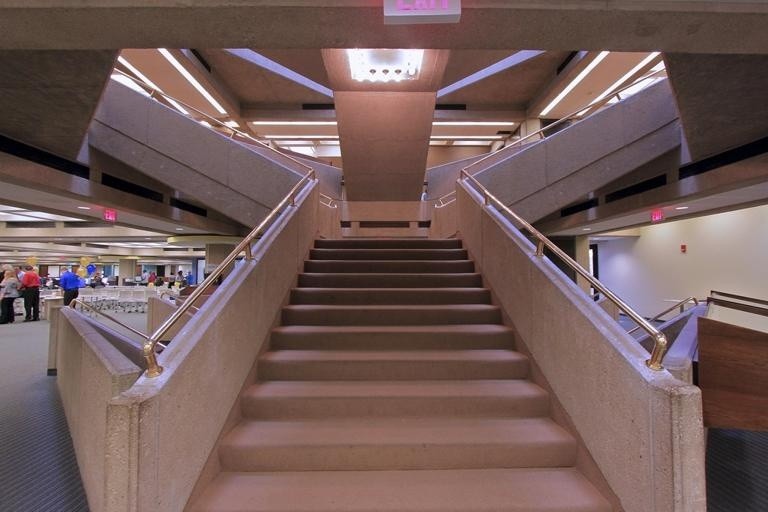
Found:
[79,285,179,313]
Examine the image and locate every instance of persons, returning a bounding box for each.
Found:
[421,189,427,201]
[0,263,194,324]
